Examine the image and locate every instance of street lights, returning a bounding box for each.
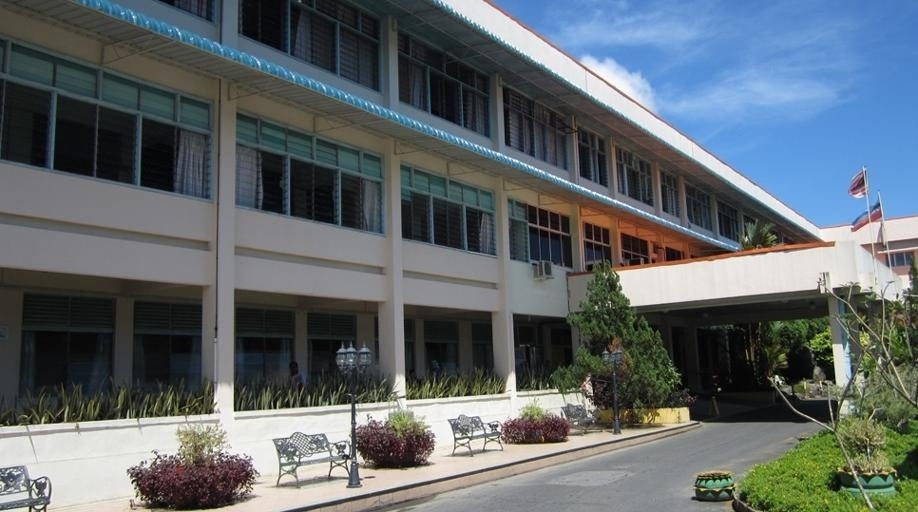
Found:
[602,345,626,436]
[336,338,373,488]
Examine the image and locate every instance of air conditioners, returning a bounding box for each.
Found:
[623,257,644,268]
[585,258,612,273]
[537,259,555,280]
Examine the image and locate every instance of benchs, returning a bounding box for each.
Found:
[561,404,597,436]
[274,431,352,488]
[449,414,506,458]
[1,465,54,511]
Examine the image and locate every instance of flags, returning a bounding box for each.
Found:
[848,169,870,198]
[851,197,881,232]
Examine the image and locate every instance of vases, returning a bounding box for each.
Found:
[692,470,736,502]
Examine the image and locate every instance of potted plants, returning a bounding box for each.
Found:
[830,374,906,496]
